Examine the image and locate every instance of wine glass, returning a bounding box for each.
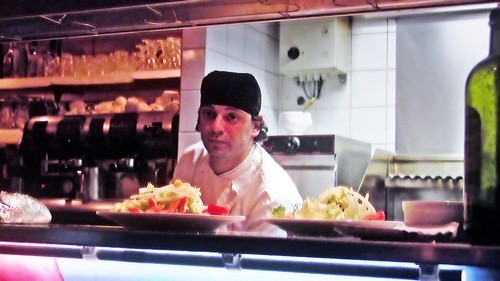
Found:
[136,36,181,71]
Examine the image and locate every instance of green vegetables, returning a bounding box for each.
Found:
[270,204,285,218]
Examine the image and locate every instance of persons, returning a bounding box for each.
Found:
[170,70,302,233]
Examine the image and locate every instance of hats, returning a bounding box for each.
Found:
[200,70,262,116]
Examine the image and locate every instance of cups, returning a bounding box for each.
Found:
[3,43,135,79]
[402,200,466,233]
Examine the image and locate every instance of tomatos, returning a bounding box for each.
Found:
[202,203,229,216]
[364,211,386,221]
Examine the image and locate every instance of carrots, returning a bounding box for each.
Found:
[128,196,188,213]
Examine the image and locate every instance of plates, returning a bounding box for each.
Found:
[96,210,246,233]
[263,217,402,238]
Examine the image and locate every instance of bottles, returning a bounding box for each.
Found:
[464,7,500,246]
